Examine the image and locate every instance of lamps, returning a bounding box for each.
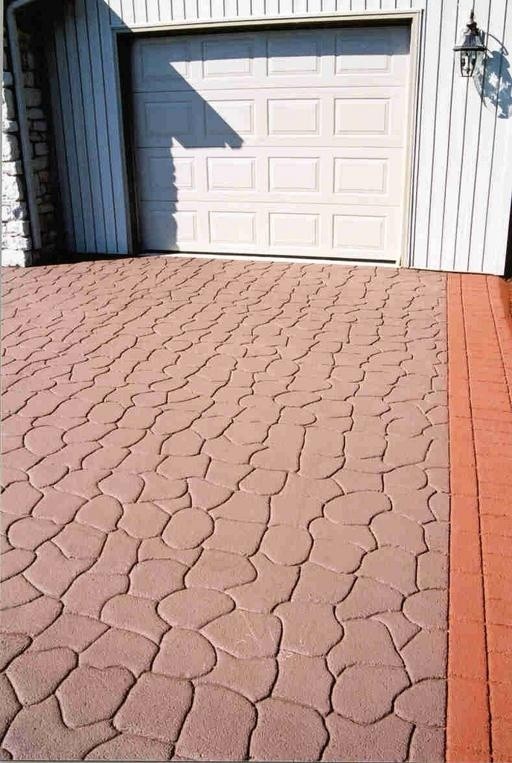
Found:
[449,8,490,81]
